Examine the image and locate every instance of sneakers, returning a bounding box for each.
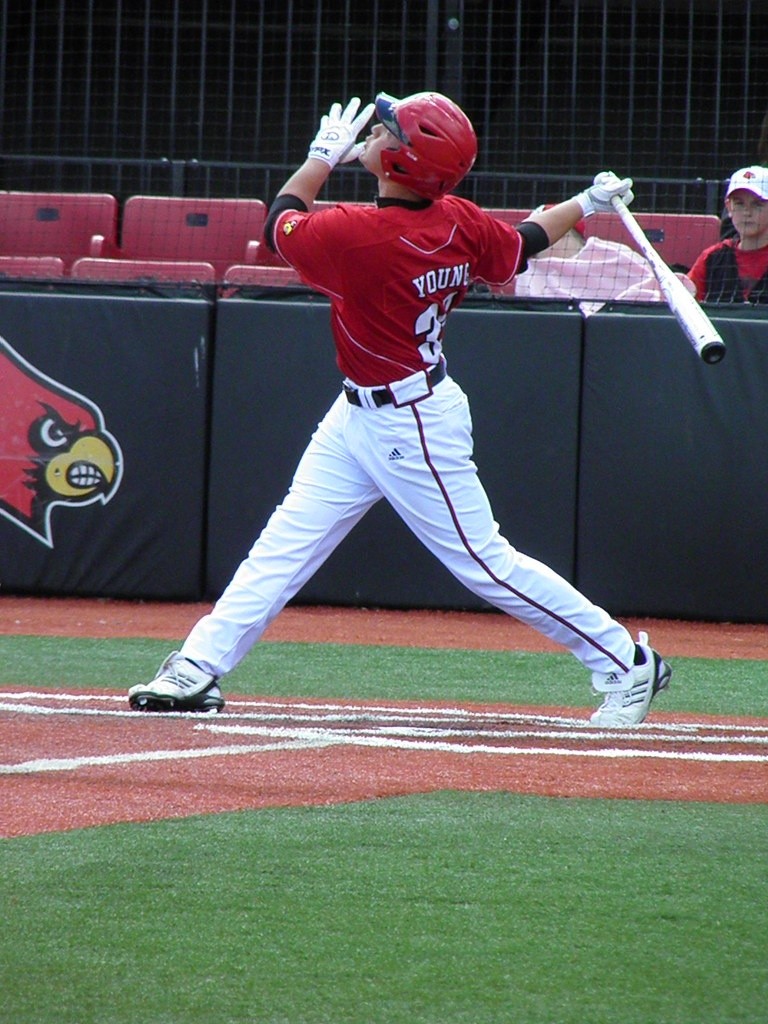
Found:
[590,630,672,724]
[128,650,226,714]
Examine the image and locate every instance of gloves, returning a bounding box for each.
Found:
[571,170,636,219]
[308,96,377,170]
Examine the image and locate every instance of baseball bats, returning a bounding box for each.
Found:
[591,170,730,364]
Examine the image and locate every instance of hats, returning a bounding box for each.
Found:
[726,165,768,201]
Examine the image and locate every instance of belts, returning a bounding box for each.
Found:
[343,360,446,409]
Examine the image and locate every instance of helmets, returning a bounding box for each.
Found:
[374,89,478,201]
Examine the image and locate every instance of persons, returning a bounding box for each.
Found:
[129,92,672,728]
[688,166,768,303]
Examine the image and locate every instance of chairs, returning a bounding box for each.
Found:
[0,192,722,312]
